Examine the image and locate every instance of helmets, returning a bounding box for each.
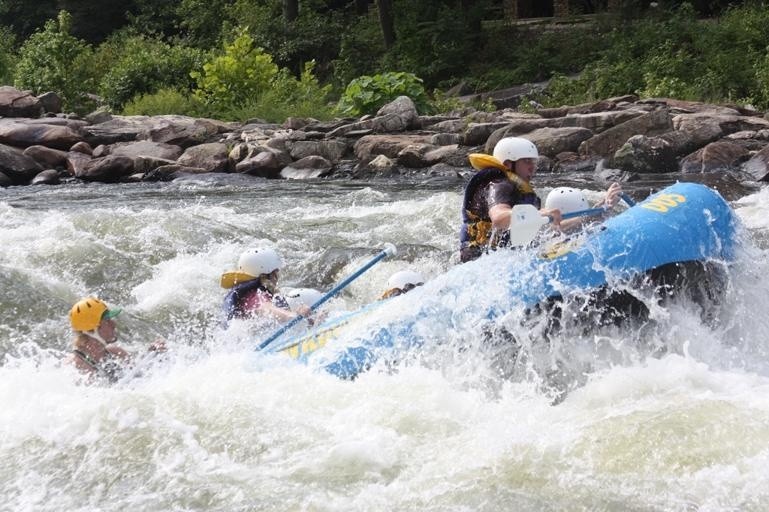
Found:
[69,296,123,331]
[491,135,540,168]
[544,186,591,215]
[238,246,281,278]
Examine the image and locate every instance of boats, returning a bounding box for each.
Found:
[244,178,735,387]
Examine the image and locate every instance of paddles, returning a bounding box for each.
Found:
[511,204,606,246]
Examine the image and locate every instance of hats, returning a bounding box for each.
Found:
[102,308,122,320]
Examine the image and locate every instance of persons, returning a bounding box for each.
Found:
[458,136,623,265]
[69,297,167,386]
[222,239,346,346]
